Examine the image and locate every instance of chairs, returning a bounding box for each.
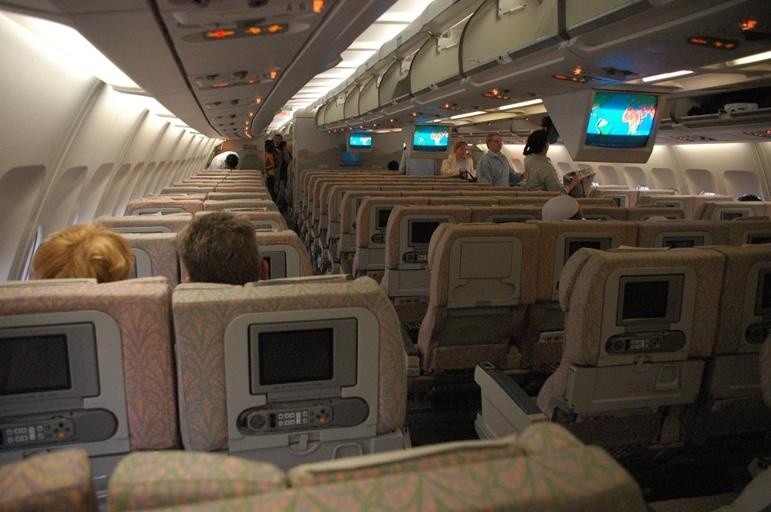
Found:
[2,275,179,501]
[169,275,409,468]
[296,169,770,380]
[657,244,771,448]
[106,450,288,512]
[2,448,98,510]
[91,168,317,282]
[472,249,726,463]
[289,422,647,511]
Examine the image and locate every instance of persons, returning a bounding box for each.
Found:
[441,142,475,180]
[523,130,580,195]
[208,151,241,169]
[263,134,293,210]
[477,134,527,187]
[737,193,761,201]
[387,160,399,171]
[29,227,133,285]
[399,149,437,177]
[175,211,270,286]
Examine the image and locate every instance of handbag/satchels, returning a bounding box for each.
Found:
[563,167,597,198]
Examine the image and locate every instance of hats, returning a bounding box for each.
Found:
[542,194,580,223]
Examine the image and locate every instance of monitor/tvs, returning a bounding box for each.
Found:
[542,89,666,163]
[0,323,117,451]
[663,237,704,250]
[109,208,301,282]
[373,207,395,244]
[401,125,452,160]
[345,131,374,153]
[493,196,749,224]
[242,317,369,432]
[744,266,771,343]
[565,238,611,264]
[748,234,771,246]
[607,275,686,353]
[406,220,448,264]
